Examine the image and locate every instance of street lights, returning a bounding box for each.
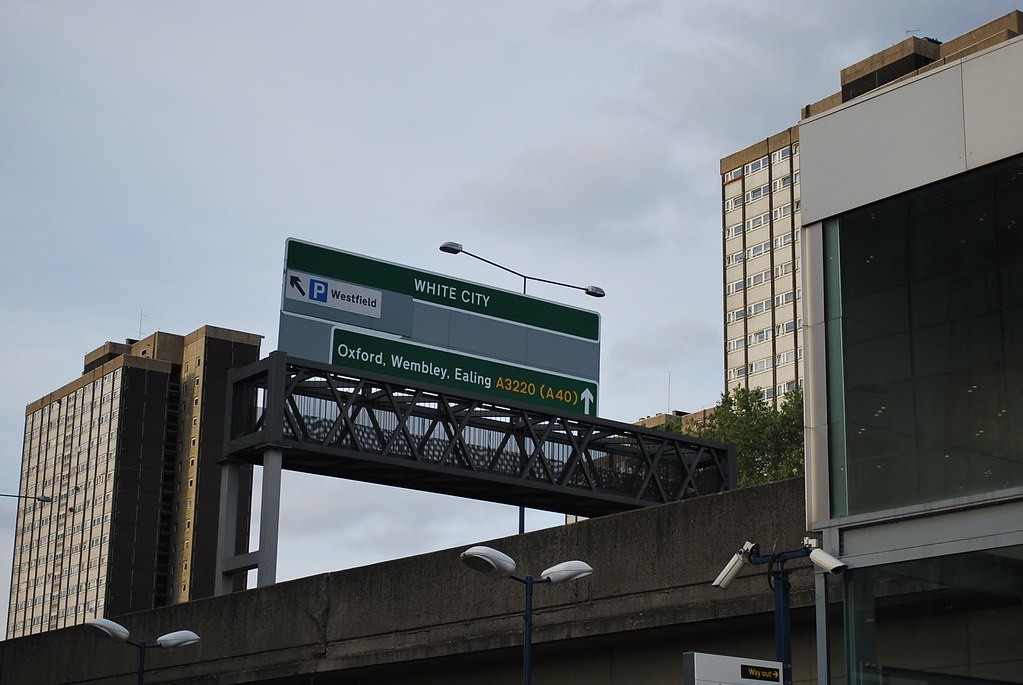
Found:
[457,545,593,684]
[88,619,202,685]
[440,241,605,534]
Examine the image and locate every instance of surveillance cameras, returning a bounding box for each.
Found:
[808,547,849,576]
[711,552,743,590]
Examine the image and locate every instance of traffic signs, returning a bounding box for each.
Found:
[277,237,601,420]
